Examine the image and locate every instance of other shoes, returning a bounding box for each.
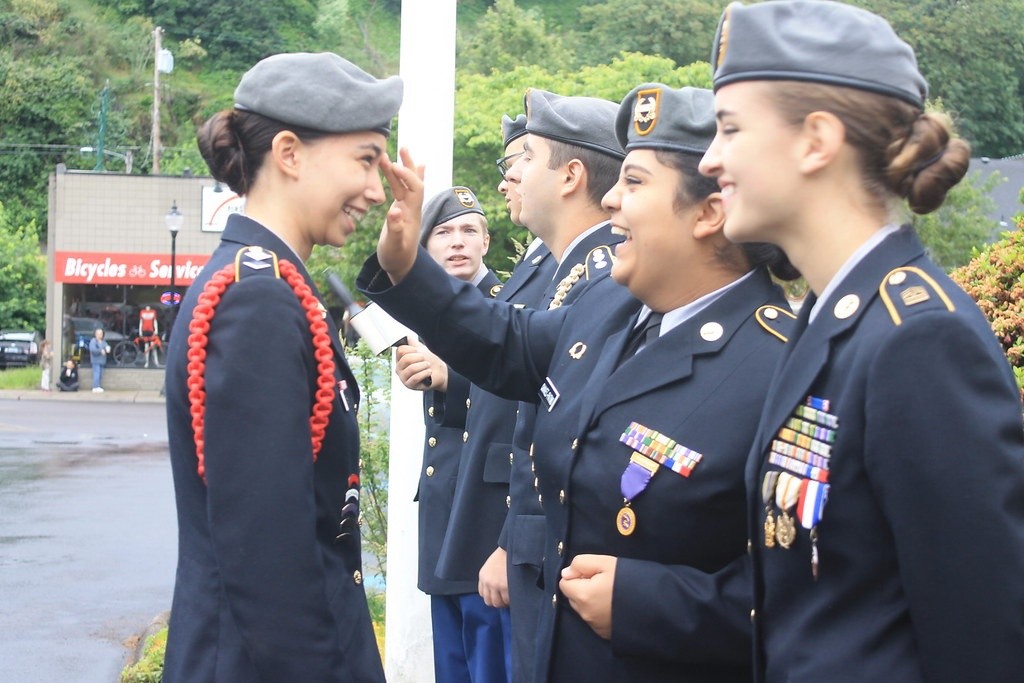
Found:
[93,387,104,392]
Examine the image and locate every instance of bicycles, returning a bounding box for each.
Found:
[113,340,170,366]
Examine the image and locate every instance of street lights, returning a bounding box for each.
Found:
[167,199,184,327]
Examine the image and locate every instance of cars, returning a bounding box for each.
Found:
[0,329,43,371]
[70,317,129,348]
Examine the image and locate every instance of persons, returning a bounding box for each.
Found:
[355,0,795,683]
[89,329,110,392]
[139,306,161,368]
[39,340,54,392]
[697,0,1024,683]
[163,52,403,683]
[56,360,79,391]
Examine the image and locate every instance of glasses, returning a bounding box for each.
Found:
[495,152,522,181]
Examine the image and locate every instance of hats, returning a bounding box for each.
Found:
[614,83,716,153]
[419,186,486,249]
[523,86,626,159]
[502,114,527,150]
[232,52,403,134]
[711,0,929,106]
